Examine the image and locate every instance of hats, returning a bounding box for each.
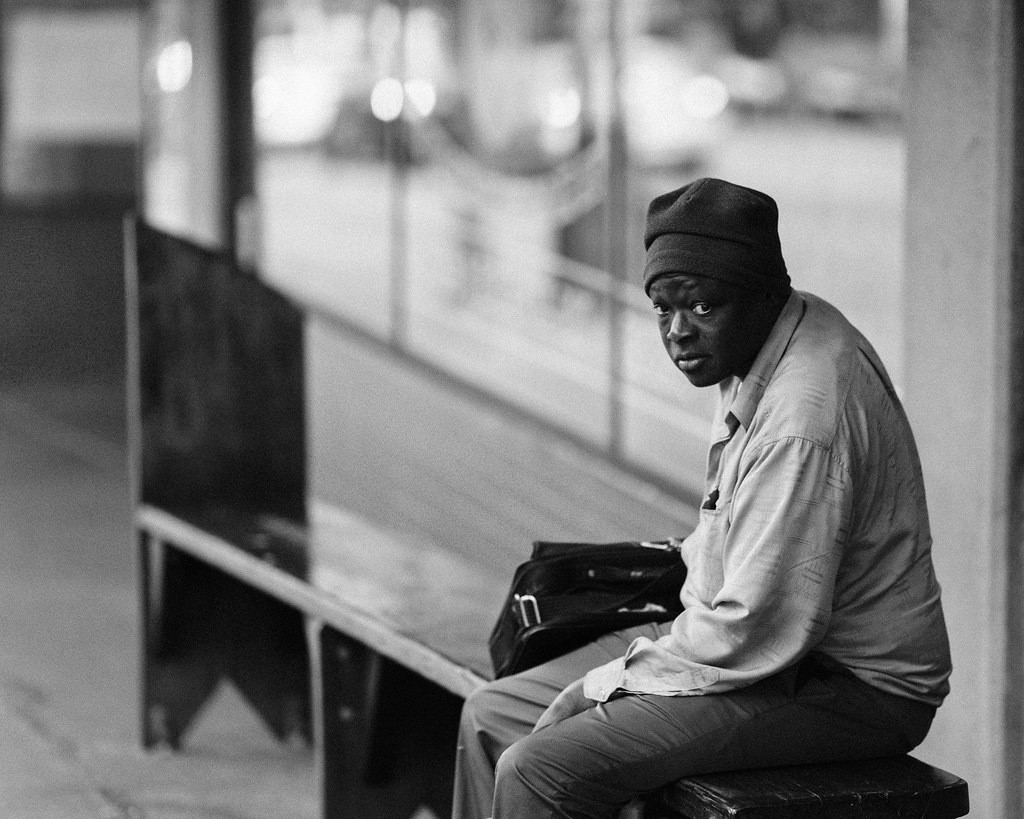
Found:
[643,178,792,295]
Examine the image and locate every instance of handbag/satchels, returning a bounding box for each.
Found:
[490,539,689,679]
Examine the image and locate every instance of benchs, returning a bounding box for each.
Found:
[133,496,971,819]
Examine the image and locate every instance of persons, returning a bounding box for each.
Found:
[451,179,953,818]
